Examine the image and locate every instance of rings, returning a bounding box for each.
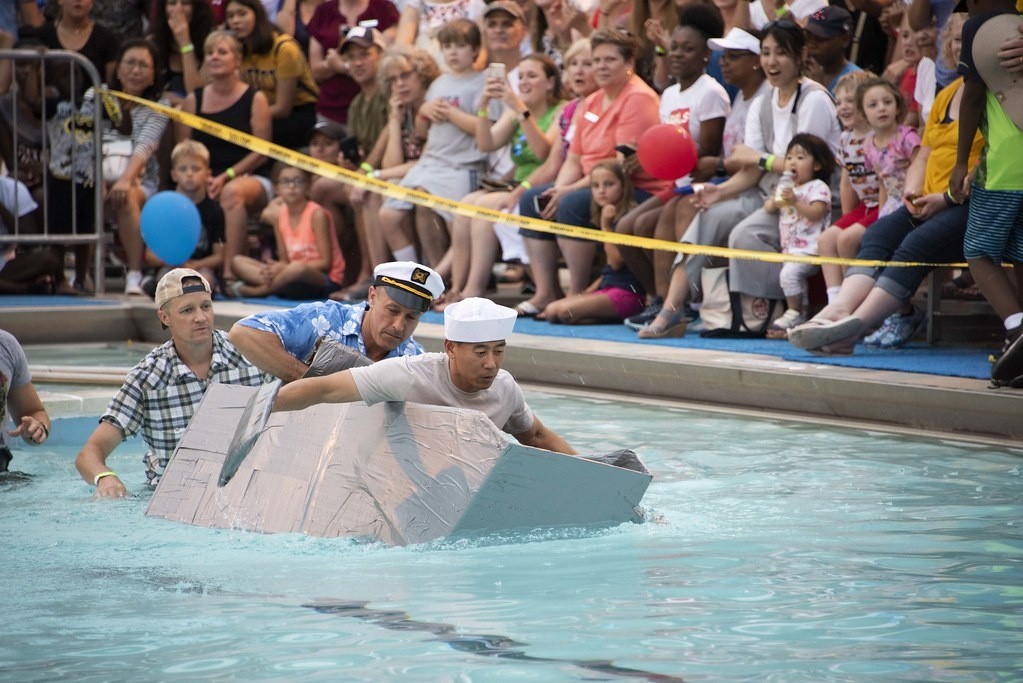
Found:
[1020,57,1023,63]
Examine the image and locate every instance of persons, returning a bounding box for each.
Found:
[75,265,281,501]
[0,328,52,472]
[0,0,1023,387]
[270,293,581,457]
[227,259,446,383]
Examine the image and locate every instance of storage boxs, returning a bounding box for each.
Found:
[144,342,655,548]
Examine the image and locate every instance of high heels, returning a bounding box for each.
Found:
[640,306,688,338]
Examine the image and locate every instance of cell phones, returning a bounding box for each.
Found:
[534,196,551,214]
[490,62,507,80]
[614,145,637,157]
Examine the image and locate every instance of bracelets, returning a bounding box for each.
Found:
[943,188,956,206]
[775,3,789,19]
[360,162,374,172]
[374,169,383,178]
[94,472,117,485]
[477,111,488,117]
[367,170,375,179]
[29,422,50,445]
[181,43,193,54]
[765,153,776,172]
[520,180,532,192]
[226,168,236,179]
[599,9,609,16]
[418,112,427,121]
[655,45,666,56]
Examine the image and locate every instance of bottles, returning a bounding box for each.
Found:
[64,252,76,287]
[402,128,422,159]
[774,168,795,208]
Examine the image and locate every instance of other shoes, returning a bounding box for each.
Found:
[140,274,156,301]
[124,269,144,295]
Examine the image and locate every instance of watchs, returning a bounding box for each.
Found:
[758,152,770,170]
[343,61,351,74]
[517,107,532,122]
[716,158,728,178]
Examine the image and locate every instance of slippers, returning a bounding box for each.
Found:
[221,278,245,299]
[787,317,863,349]
[514,299,543,318]
[806,343,854,356]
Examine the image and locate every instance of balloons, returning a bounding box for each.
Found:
[139,190,201,265]
[638,121,698,181]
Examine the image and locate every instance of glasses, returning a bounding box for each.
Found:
[278,176,307,187]
[122,58,153,70]
[720,52,754,61]
[384,69,415,81]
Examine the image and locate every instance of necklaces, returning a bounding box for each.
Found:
[62,24,86,36]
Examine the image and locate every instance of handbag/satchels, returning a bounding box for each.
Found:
[696,265,783,338]
[98,138,146,182]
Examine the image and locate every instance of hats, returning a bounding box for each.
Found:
[444,297,518,344]
[339,25,387,54]
[373,261,445,313]
[155,268,212,330]
[709,27,761,56]
[801,5,852,36]
[484,0,528,25]
[309,121,347,142]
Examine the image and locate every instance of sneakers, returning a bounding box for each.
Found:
[988,319,1023,390]
[773,307,804,331]
[625,295,693,328]
[863,304,927,351]
